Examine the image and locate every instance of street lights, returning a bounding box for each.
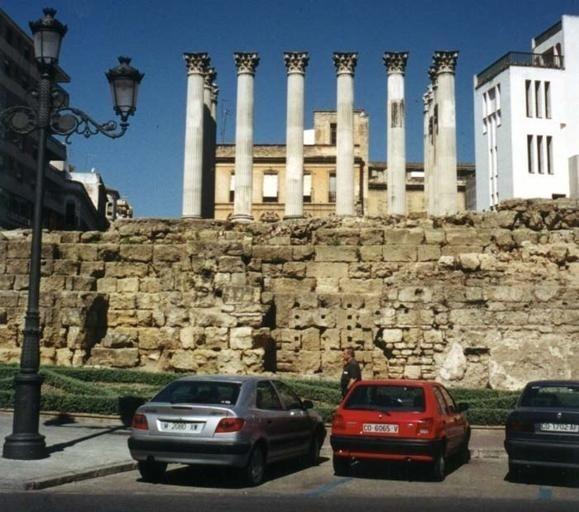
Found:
[0,8,147,459]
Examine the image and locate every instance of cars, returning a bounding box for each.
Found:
[502,380,578,475]
[128,373,328,486]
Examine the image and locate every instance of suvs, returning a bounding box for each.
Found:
[329,378,472,481]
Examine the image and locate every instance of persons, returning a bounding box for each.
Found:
[341,349,361,400]
